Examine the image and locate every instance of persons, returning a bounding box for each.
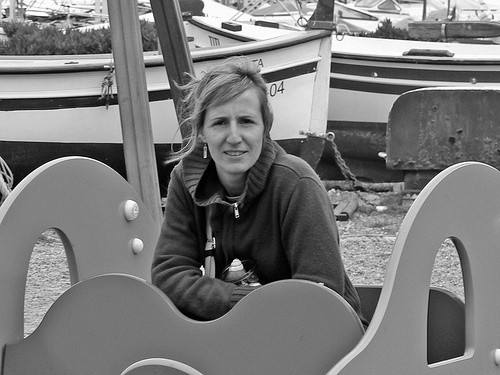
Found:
[150,56,370,331]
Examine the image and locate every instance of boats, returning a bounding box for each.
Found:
[0,0,499,163]
[0,0,338,208]
